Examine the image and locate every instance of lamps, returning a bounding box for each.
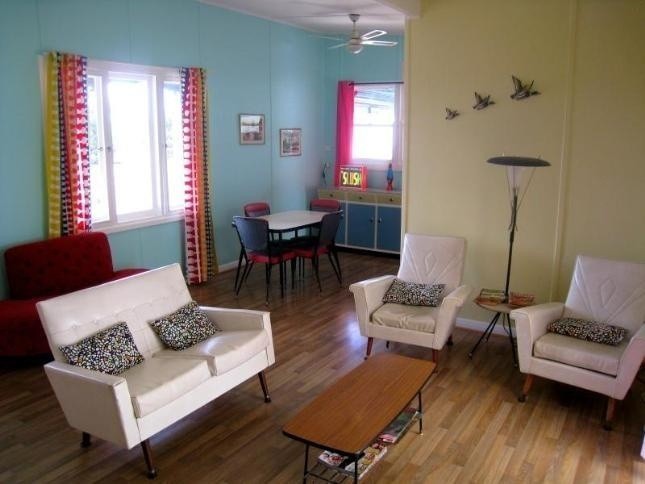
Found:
[468,149,552,366]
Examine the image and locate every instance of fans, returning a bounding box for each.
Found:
[309,15,398,55]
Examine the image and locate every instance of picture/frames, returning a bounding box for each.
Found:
[280,127,303,157]
[238,113,266,146]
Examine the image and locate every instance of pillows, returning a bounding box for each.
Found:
[56,322,144,379]
[147,299,219,350]
[382,278,447,307]
[548,317,625,346]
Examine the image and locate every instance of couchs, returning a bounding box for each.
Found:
[34,261,277,478]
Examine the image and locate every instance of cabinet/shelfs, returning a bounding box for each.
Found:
[345,203,403,251]
[325,200,347,248]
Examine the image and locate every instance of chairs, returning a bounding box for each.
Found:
[295,209,342,290]
[1,230,151,364]
[244,202,292,284]
[509,252,645,431]
[233,213,293,296]
[348,233,472,374]
[292,200,340,279]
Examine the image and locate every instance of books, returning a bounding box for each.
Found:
[317,442,387,480]
[377,406,418,443]
